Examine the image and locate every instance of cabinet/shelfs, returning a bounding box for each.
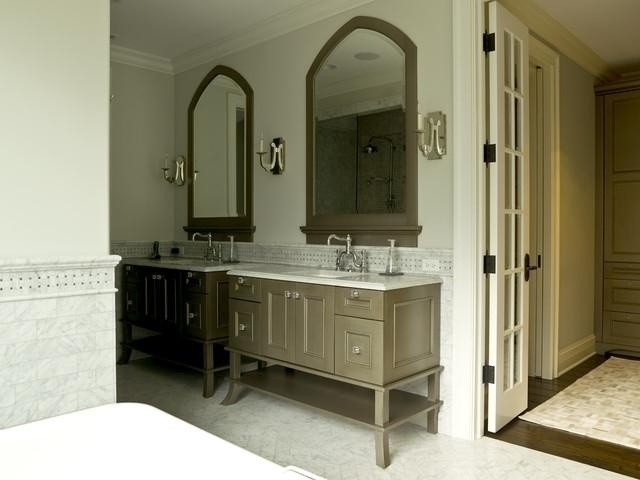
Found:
[142,264,176,327]
[259,278,335,378]
[117,260,145,323]
[223,276,263,357]
[589,66,640,362]
[171,270,231,341]
[331,281,443,388]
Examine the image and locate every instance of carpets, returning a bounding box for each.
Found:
[519,350,640,452]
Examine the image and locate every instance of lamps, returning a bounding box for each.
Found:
[257,131,287,177]
[161,152,187,188]
[415,103,447,162]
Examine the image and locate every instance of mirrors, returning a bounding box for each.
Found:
[185,62,257,233]
[302,11,422,236]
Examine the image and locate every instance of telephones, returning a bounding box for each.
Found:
[149,241,161,260]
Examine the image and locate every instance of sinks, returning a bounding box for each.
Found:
[158,259,205,265]
[285,270,363,279]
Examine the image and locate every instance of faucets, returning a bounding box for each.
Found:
[327,232,360,268]
[191,232,217,260]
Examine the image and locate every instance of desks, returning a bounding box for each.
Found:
[0,401,315,480]
